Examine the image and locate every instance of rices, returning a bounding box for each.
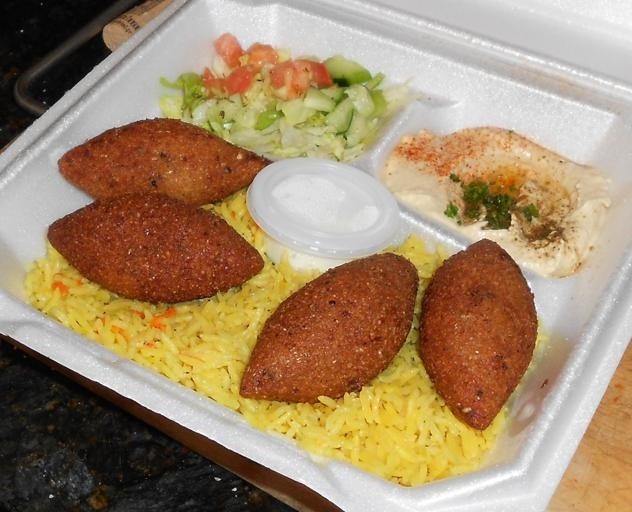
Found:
[22,186,549,486]
